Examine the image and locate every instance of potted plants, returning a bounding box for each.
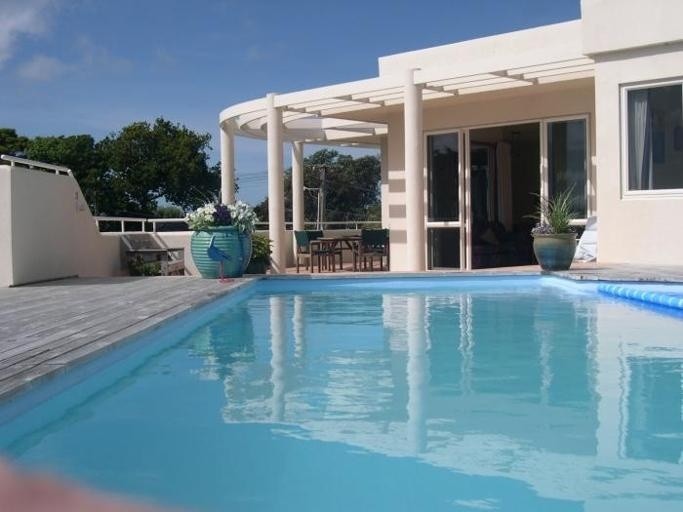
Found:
[520,181,585,271]
[245,233,276,275]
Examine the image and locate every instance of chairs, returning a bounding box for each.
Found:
[470,222,508,267]
[293,228,389,273]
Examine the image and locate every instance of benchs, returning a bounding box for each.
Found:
[120,233,185,276]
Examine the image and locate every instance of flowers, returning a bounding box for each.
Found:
[180,182,261,237]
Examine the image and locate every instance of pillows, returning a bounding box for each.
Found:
[480,229,497,243]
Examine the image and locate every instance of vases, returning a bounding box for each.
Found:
[190,225,252,280]
